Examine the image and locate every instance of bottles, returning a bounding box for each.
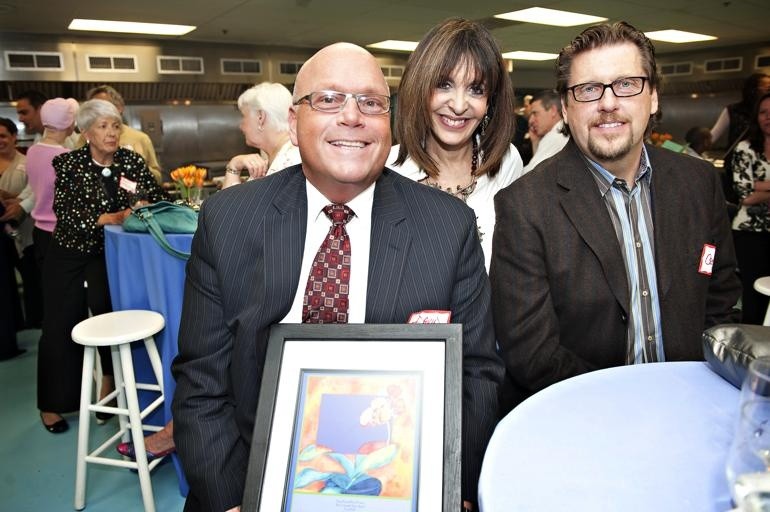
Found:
[720,354,770,512]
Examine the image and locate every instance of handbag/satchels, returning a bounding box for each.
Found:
[701,324,770,397]
[122,200,198,260]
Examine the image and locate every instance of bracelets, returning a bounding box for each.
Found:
[223,167,240,176]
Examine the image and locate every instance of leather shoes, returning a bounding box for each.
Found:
[0,348,26,360]
[117,440,179,462]
[40,411,69,434]
[96,397,117,420]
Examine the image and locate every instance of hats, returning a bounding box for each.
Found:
[40,96,79,132]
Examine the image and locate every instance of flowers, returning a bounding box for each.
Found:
[650,131,674,147]
[170,163,207,207]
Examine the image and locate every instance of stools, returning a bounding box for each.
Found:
[753,275,770,327]
[70,307,165,512]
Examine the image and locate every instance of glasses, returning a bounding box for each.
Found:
[565,75,653,103]
[294,88,396,117]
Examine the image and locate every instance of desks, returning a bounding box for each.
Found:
[478,361,770,511]
[102,225,196,497]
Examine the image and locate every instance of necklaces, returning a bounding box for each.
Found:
[421,137,478,203]
[90,156,113,178]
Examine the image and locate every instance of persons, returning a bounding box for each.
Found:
[719,93,770,325]
[684,127,712,160]
[112,81,302,461]
[521,94,533,120]
[487,20,743,400]
[520,88,571,176]
[711,73,770,155]
[382,15,523,274]
[0,83,163,434]
[170,40,511,512]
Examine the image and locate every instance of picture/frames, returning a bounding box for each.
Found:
[241,322,463,512]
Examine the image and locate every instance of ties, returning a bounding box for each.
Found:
[301,204,355,323]
